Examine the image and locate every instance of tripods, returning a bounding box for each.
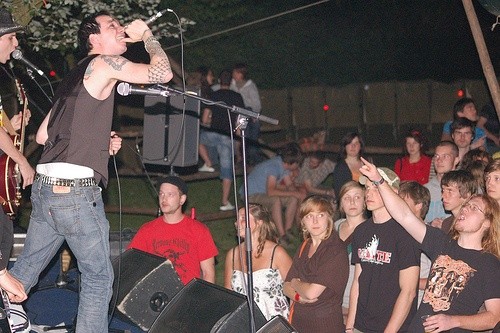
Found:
[34,252,80,292]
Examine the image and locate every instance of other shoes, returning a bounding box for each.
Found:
[198,163,215,173]
[219,201,235,210]
[276,228,296,246]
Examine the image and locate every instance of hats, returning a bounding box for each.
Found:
[161,176,189,193]
[0,5,22,37]
[359,167,404,190]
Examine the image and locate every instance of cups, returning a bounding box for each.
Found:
[420,315,430,324]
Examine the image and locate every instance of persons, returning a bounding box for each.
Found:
[393,97,500,205]
[427,169,478,234]
[423,141,461,224]
[0,252,28,303]
[399,180,431,308]
[0,6,36,267]
[333,181,367,326]
[9,11,174,333]
[283,195,348,333]
[359,156,500,333]
[346,166,419,333]
[129,175,219,288]
[225,203,295,322]
[196,63,307,244]
[291,132,363,234]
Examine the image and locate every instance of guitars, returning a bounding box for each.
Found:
[0,77,28,220]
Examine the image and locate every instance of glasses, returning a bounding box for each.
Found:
[463,202,487,216]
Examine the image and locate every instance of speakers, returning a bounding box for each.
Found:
[148,277,266,333]
[142,84,202,167]
[254,315,298,333]
[111,248,185,330]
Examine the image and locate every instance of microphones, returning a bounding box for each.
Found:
[12,50,45,75]
[145,9,168,25]
[116,81,169,97]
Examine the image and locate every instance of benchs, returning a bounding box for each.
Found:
[21,120,295,245]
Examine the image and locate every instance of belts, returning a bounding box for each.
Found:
[34,172,96,187]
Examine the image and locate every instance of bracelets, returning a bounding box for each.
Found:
[325,190,328,196]
[295,293,299,301]
[374,178,384,186]
[344,328,354,333]
[144,38,162,54]
